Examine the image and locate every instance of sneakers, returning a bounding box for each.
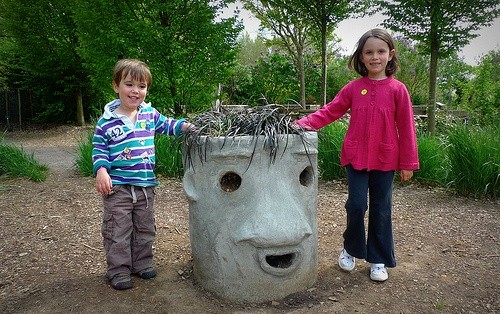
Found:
[338,249,356,272]
[111,273,133,290]
[370,263,389,282]
[139,268,158,279]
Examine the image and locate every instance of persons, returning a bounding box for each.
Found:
[91,59,197,290]
[288,28,420,281]
